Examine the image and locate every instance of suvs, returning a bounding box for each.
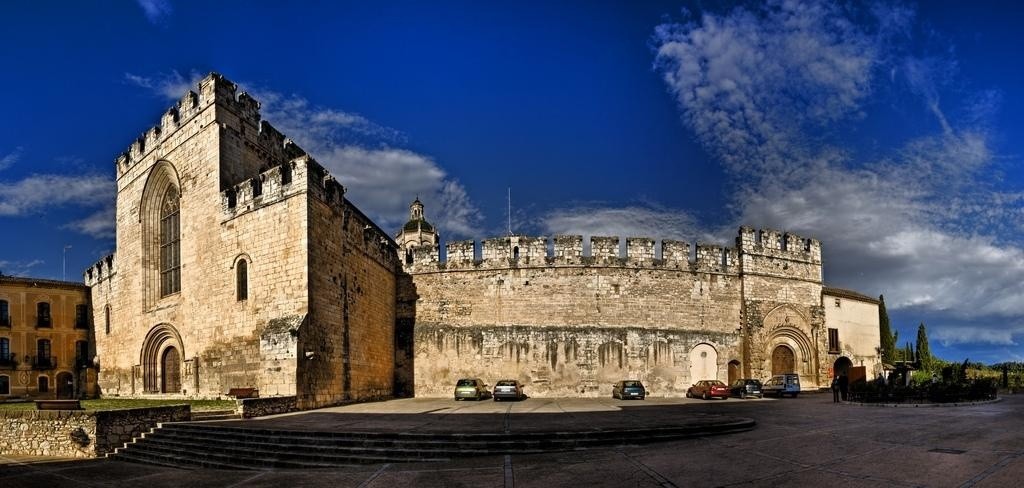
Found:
[730,379,764,399]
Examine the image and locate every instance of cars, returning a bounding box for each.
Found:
[493,381,523,401]
[613,380,645,401]
[686,380,732,400]
[455,378,488,401]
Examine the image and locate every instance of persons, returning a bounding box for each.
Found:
[831,370,849,404]
[877,372,884,384]
[932,374,938,386]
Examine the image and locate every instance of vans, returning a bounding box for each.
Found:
[761,374,800,397]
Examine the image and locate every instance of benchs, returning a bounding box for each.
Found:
[225,387,255,398]
[34,399,80,410]
